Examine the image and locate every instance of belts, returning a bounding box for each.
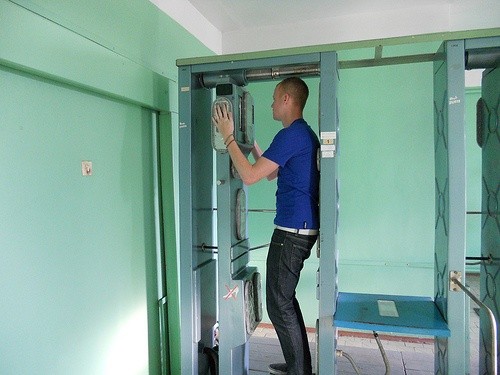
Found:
[274,224,319,235]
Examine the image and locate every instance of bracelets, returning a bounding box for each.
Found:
[224,133,235,148]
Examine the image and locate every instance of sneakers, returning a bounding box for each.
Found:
[267,363,289,375]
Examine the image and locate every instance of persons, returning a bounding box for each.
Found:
[211,76,320,375]
